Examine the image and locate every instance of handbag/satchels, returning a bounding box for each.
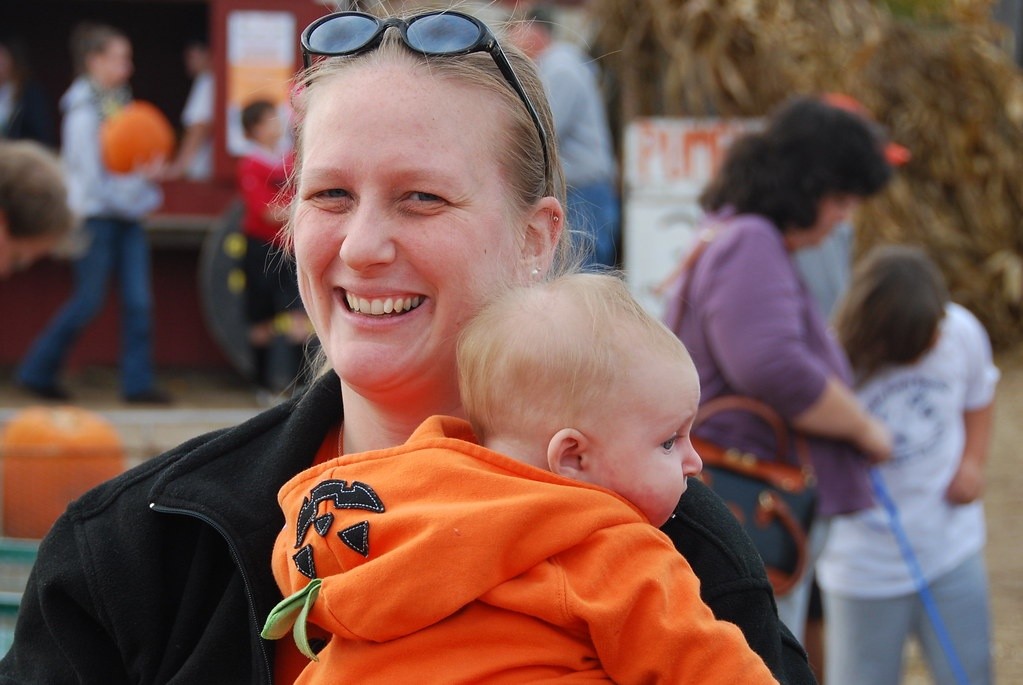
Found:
[684,398,818,598]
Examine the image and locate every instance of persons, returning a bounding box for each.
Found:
[654,94,892,649]
[815,244,1002,685]
[505,10,623,273]
[0,26,815,685]
[261,275,782,685]
[0,22,293,410]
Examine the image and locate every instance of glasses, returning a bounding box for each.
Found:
[302,8,550,199]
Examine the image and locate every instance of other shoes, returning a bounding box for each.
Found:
[21,378,75,401]
[255,384,300,411]
[122,387,172,405]
